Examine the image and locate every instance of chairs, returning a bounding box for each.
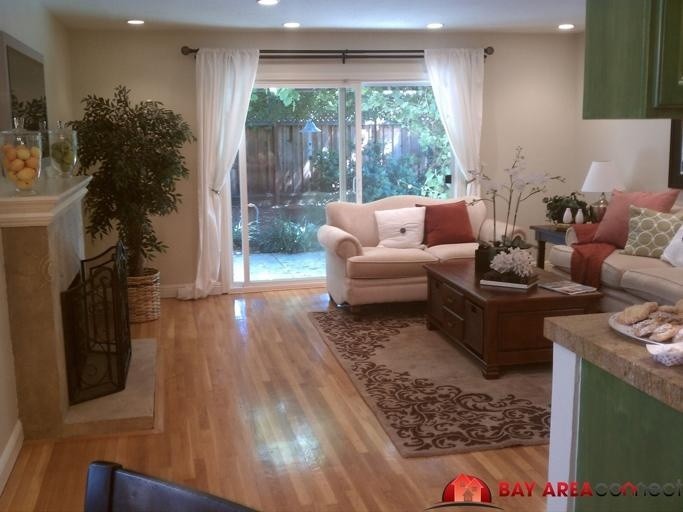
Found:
[583,1,683,184]
[82,461,257,511]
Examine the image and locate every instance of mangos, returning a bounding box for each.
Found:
[0,144,40,190]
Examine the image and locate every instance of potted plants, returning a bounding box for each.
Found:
[66,83,196,323]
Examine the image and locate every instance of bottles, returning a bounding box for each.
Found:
[48,120,77,177]
[563,208,572,224]
[0,117,41,196]
[575,208,584,224]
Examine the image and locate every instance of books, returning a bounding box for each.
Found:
[537,280,597,296]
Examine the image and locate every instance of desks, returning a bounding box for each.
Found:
[545,312,683,512]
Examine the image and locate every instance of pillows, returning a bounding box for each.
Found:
[374,207,427,250]
[416,203,475,245]
[619,206,681,259]
[593,190,680,248]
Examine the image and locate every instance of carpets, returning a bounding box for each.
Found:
[309,308,551,458]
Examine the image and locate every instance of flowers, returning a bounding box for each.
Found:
[465,144,568,250]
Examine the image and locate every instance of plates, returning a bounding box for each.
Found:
[608,312,661,345]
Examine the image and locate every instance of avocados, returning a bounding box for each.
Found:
[51,140,73,173]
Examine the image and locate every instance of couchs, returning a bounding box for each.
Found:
[543,206,683,312]
[316,194,526,313]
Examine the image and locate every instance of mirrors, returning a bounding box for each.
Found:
[0,31,51,169]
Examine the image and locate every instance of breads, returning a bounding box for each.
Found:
[616,298,683,344]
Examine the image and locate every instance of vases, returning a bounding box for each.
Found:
[476,249,508,277]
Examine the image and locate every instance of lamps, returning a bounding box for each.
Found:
[582,162,625,224]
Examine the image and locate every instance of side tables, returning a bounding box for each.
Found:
[530,225,567,270]
